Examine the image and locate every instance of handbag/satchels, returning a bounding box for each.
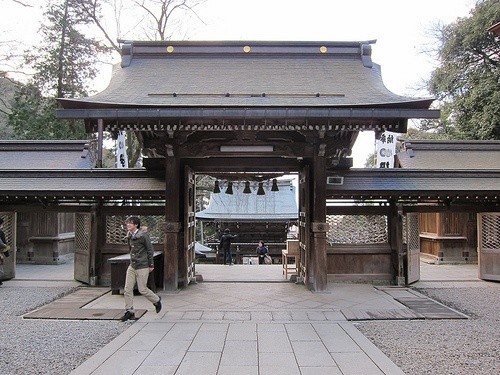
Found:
[264,254,272,264]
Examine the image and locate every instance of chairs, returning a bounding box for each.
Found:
[282,240,300,279]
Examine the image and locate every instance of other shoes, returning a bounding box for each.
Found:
[121,310,135,322]
[153,295,162,313]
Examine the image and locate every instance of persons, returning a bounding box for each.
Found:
[119,217,161,321]
[257,240,268,264]
[0,218,10,285]
[217,228,237,265]
